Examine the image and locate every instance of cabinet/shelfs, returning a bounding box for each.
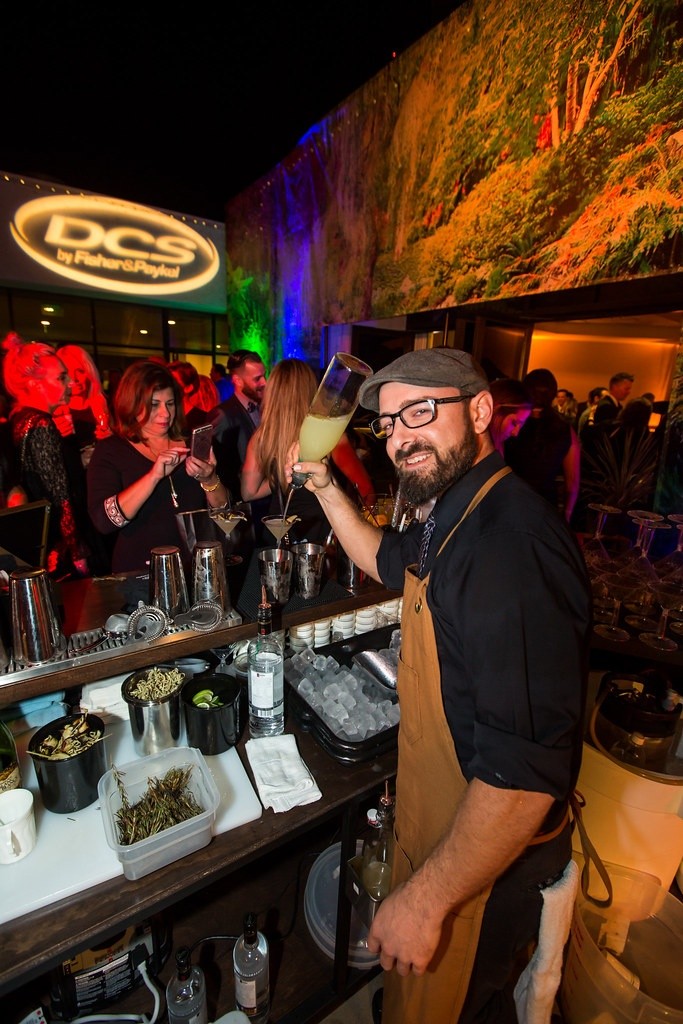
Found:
[0,570,405,1024]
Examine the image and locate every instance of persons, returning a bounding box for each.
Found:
[508,368,581,522]
[284,347,592,1024]
[0,331,373,585]
[482,378,533,472]
[594,373,635,442]
[552,387,666,535]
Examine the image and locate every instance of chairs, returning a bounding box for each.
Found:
[0,499,51,569]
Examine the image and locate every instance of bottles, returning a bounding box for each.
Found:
[246,604,286,739]
[165,951,209,1024]
[232,921,272,1024]
[288,351,373,491]
[362,795,395,899]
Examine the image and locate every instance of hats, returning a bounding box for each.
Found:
[357,348,490,411]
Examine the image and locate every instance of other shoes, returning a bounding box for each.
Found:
[371,986,384,1024]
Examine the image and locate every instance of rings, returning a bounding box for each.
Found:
[196,473,200,478]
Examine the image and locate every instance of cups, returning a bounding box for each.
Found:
[335,539,375,590]
[122,664,187,759]
[257,549,295,607]
[0,788,37,865]
[149,546,190,619]
[290,543,326,600]
[8,566,66,667]
[27,713,109,814]
[180,672,242,756]
[192,541,232,618]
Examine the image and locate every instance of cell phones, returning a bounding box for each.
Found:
[191,424,214,466]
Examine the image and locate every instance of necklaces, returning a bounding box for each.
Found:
[139,433,170,459]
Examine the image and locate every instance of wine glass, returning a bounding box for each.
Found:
[577,503,683,652]
[261,515,298,547]
[210,510,245,566]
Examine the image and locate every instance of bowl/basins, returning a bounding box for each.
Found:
[96,745,221,881]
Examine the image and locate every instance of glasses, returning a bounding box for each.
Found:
[368,393,477,438]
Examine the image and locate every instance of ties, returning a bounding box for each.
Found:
[416,508,437,579]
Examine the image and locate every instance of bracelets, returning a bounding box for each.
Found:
[199,475,220,493]
